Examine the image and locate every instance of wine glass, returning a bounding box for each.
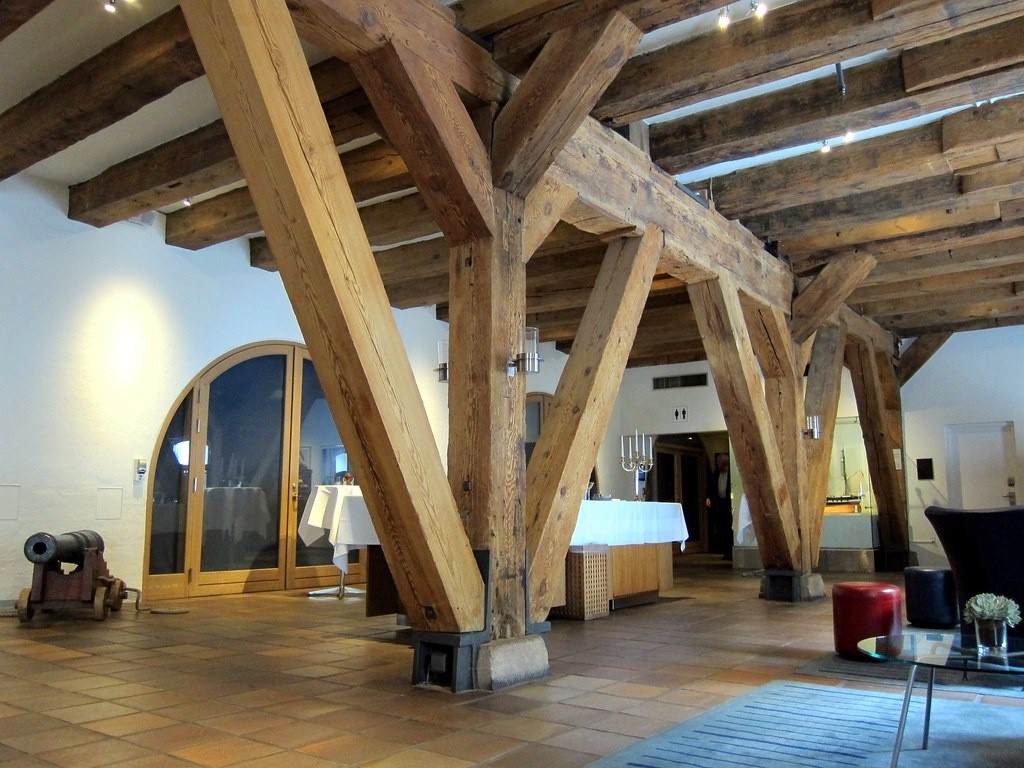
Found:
[585,482,594,500]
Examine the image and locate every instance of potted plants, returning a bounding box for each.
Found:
[964,593,1021,649]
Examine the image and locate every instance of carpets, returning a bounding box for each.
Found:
[792,653,1024,698]
[589,678,1024,768]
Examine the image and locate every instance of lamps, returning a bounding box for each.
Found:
[717,4,731,31]
[619,429,653,501]
[745,0,768,18]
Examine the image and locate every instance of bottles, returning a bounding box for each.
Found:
[642,487,648,501]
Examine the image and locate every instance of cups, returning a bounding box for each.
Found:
[342,471,354,485]
[335,475,342,484]
[593,494,602,498]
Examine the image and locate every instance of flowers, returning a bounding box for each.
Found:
[343,473,355,485]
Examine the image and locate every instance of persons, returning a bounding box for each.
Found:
[706,454,732,561]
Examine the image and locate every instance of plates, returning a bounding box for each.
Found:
[591,496,611,500]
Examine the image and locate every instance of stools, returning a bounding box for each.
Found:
[904,566,958,629]
[832,582,902,661]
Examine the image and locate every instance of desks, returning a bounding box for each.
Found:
[857,630,1024,768]
[203,487,271,531]
[298,484,381,599]
[570,500,689,610]
